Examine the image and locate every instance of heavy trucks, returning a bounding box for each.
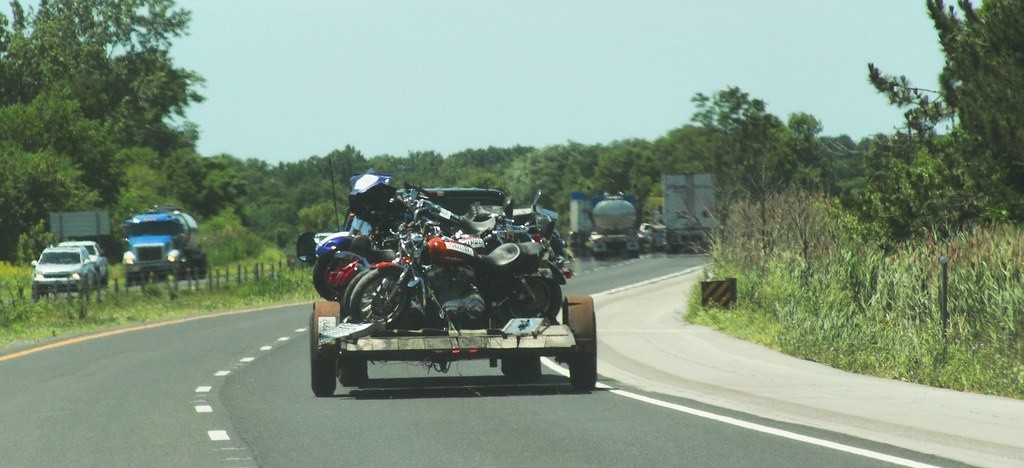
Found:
[586,189,641,260]
[660,170,722,252]
[122,204,208,288]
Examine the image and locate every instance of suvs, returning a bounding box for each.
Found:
[59,241,109,289]
[29,245,97,301]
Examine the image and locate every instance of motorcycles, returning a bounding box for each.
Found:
[310,171,574,332]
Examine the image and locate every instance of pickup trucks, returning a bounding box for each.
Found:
[308,186,597,396]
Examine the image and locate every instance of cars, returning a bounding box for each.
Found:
[638,223,654,244]
